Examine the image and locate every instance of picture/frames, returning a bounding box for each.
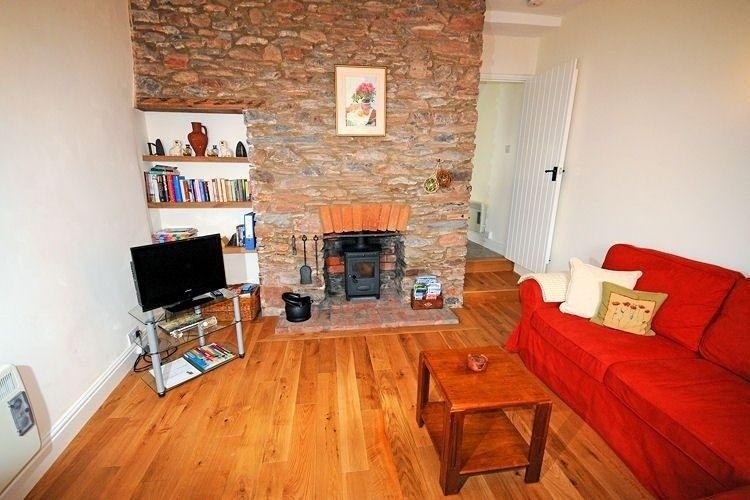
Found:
[335,64,388,138]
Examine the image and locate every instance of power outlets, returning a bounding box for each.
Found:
[127,325,142,344]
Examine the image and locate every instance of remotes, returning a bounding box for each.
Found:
[211,290,223,296]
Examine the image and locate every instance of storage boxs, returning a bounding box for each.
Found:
[410,289,443,309]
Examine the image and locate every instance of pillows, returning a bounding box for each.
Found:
[589,281,669,336]
[560,256,644,319]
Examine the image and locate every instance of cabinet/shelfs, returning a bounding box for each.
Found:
[128,288,244,397]
[135,102,256,253]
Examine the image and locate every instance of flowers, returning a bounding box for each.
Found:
[352,82,376,103]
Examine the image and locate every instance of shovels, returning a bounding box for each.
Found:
[300,235,312,284]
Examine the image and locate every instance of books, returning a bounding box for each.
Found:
[152,228,198,244]
[144,164,251,205]
[184,342,237,372]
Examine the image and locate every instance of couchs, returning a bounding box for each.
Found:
[503,243,750,500]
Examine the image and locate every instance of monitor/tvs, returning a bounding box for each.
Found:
[130,233,228,313]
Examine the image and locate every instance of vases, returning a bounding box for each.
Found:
[361,101,370,115]
[187,122,208,156]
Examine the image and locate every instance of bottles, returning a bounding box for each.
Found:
[185,144,192,156]
[211,145,219,156]
